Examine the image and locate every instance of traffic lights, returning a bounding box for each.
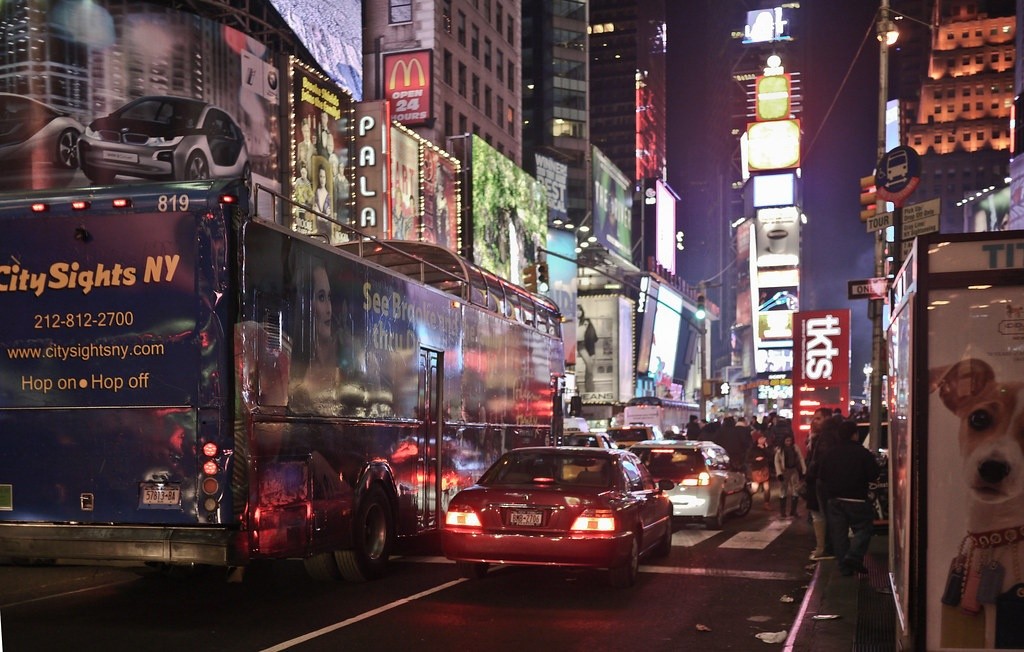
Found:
[717,380,731,395]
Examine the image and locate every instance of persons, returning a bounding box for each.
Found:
[686,415,702,440]
[296,109,350,239]
[773,406,880,573]
[576,305,598,392]
[235,256,408,492]
[434,177,451,247]
[700,412,793,511]
[392,189,418,240]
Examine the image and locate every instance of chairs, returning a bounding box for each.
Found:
[577,470,602,484]
[503,461,559,482]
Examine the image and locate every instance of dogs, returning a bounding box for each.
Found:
[938,359,1024,651]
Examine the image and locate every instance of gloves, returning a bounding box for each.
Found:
[778,475,784,481]
[799,473,804,481]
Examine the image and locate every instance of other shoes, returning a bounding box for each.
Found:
[810,550,836,559]
[764,504,774,511]
[843,558,869,574]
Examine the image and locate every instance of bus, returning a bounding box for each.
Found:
[622,396,700,440]
[1,177,568,587]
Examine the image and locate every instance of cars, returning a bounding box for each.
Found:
[443,444,675,588]
[607,426,658,453]
[562,427,618,482]
[75,95,253,188]
[627,440,753,530]
[0,92,88,175]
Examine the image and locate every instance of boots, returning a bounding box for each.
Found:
[790,496,801,517]
[779,496,787,519]
